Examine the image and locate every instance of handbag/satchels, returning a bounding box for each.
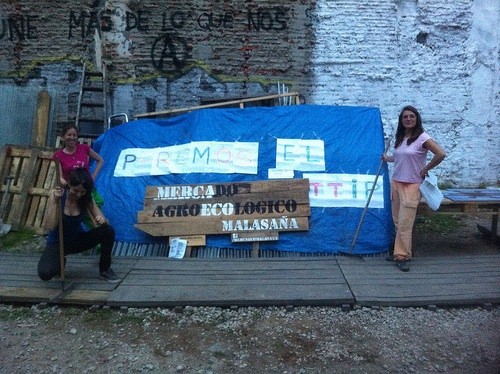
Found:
[419,178,443,211]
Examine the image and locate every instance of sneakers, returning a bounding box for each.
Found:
[53,275,61,280]
[386,255,411,261]
[394,261,409,271]
[100,268,120,282]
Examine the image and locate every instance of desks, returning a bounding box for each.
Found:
[416,189,500,248]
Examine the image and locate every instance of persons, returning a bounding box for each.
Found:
[53,124,104,186]
[381,105,446,271]
[37,167,120,283]
